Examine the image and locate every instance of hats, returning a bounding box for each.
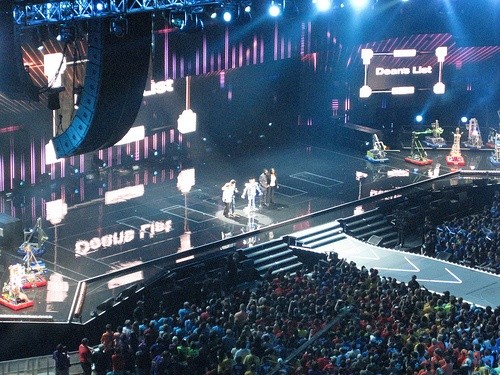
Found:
[461,349,467,355]
[206,306,210,311]
[183,302,189,307]
[465,358,471,366]
[82,338,88,345]
[431,338,436,342]
[366,325,372,331]
[277,358,283,363]
[458,321,464,327]
[443,291,450,295]
[330,356,338,359]
[172,336,178,342]
[357,354,362,358]
[433,361,439,367]
[56,344,66,350]
[226,328,231,334]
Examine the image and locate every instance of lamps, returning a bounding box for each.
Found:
[150,148,157,156]
[68,165,78,178]
[13,178,24,192]
[93,155,106,175]
[111,16,128,37]
[240,1,250,12]
[59,23,76,43]
[203,5,217,18]
[169,7,187,29]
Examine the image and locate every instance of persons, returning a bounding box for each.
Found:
[53,344,70,375]
[78,167,500,375]
